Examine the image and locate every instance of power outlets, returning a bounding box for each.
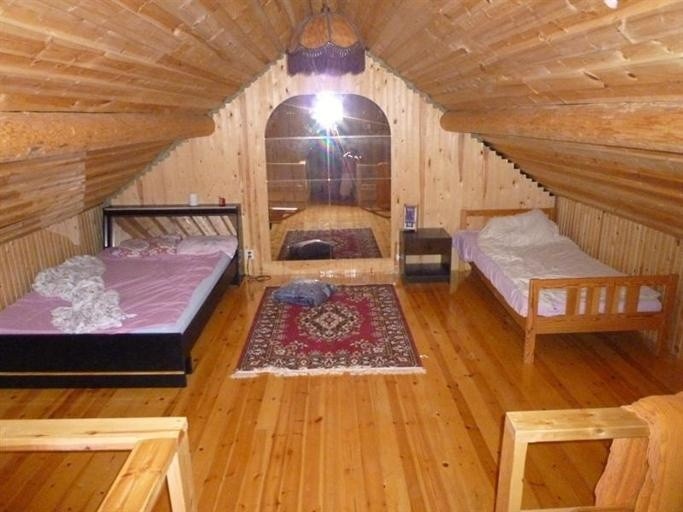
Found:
[246,251,253,260]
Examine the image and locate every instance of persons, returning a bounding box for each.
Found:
[304,123,355,202]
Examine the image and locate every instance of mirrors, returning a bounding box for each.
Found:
[265,92,393,260]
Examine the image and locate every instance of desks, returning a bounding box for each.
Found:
[398,226,453,284]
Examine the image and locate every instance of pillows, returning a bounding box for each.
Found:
[109,233,238,261]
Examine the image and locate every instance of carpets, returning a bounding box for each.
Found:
[277,226,383,259]
[229,282,428,384]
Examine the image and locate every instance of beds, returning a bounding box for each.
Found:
[457,206,680,364]
[0,203,244,391]
